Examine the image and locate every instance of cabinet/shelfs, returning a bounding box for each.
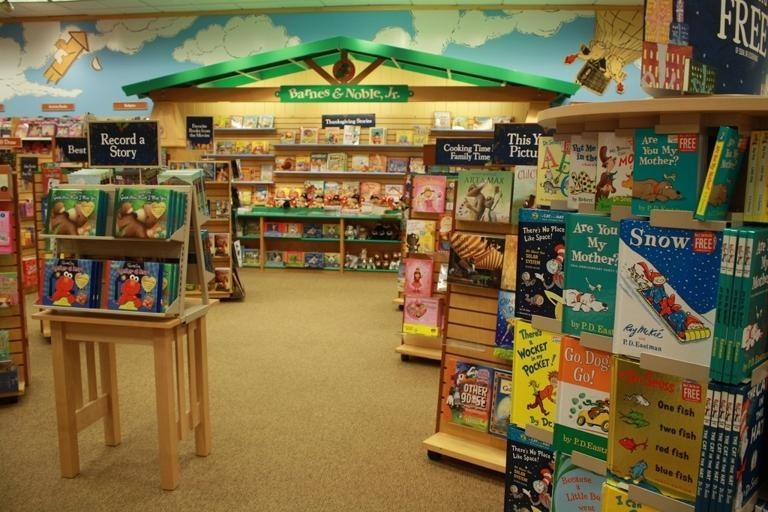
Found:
[201,128,423,273]
[168,160,234,298]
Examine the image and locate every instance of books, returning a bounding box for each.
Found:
[438,353,493,432]
[0,115,204,394]
[486,366,521,439]
[343,182,404,198]
[213,115,274,153]
[398,164,504,341]
[273,125,429,172]
[203,158,273,290]
[267,180,343,268]
[434,111,503,130]
[501,115,768,511]
